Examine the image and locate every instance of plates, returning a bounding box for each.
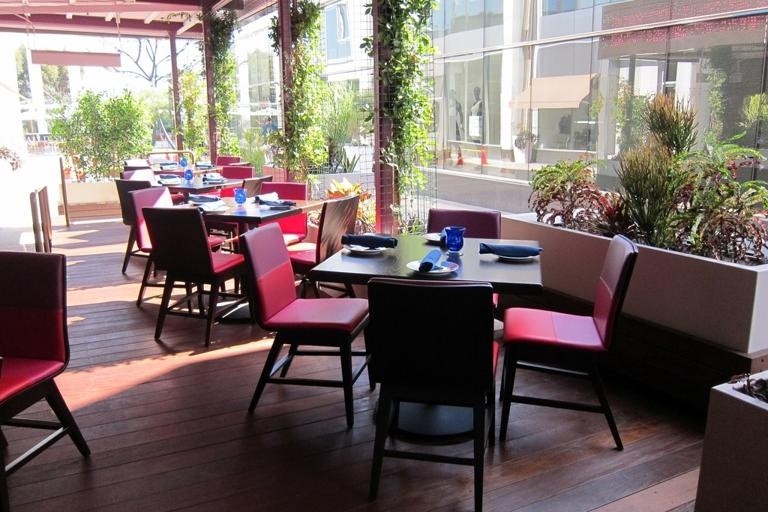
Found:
[161,173,224,186]
[499,254,540,262]
[407,260,459,277]
[344,244,387,255]
[184,199,289,212]
[421,232,441,242]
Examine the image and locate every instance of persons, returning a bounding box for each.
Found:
[449,89,463,141]
[471,86,483,144]
[263,116,278,135]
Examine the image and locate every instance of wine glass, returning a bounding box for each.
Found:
[234,188,248,209]
[180,157,188,168]
[185,169,193,184]
[445,225,466,256]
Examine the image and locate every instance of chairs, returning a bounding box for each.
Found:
[428,210,502,310]
[499,234,641,451]
[0,252,90,512]
[240,222,368,428]
[363,277,499,512]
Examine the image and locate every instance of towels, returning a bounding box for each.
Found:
[340,233,397,249]
[439,227,448,247]
[480,242,544,258]
[417,250,443,271]
